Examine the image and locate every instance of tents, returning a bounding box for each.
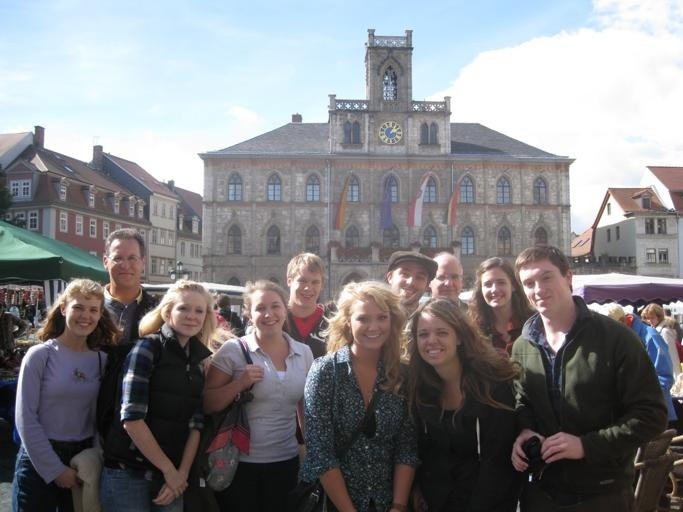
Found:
[572,271,683,316]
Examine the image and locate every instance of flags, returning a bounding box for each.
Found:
[334,172,353,228]
[378,171,396,229]
[444,180,462,226]
[406,171,430,227]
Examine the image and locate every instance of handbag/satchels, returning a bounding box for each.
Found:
[206,338,256,492]
[297,354,384,512]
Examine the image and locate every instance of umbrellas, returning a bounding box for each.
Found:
[0,215,112,310]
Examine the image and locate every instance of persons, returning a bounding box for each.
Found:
[214,294,244,336]
[595,302,681,428]
[10,304,21,318]
[468,258,535,357]
[0,302,24,352]
[664,307,683,342]
[282,251,336,359]
[25,297,37,328]
[429,251,470,310]
[638,303,683,396]
[295,281,424,511]
[202,281,315,512]
[383,250,438,318]
[100,228,160,361]
[100,282,217,512]
[509,245,669,511]
[9,279,106,512]
[405,296,520,512]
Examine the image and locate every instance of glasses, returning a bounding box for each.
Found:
[106,254,143,265]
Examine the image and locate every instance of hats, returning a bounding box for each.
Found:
[387,251,437,282]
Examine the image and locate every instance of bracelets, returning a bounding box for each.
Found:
[391,503,408,512]
